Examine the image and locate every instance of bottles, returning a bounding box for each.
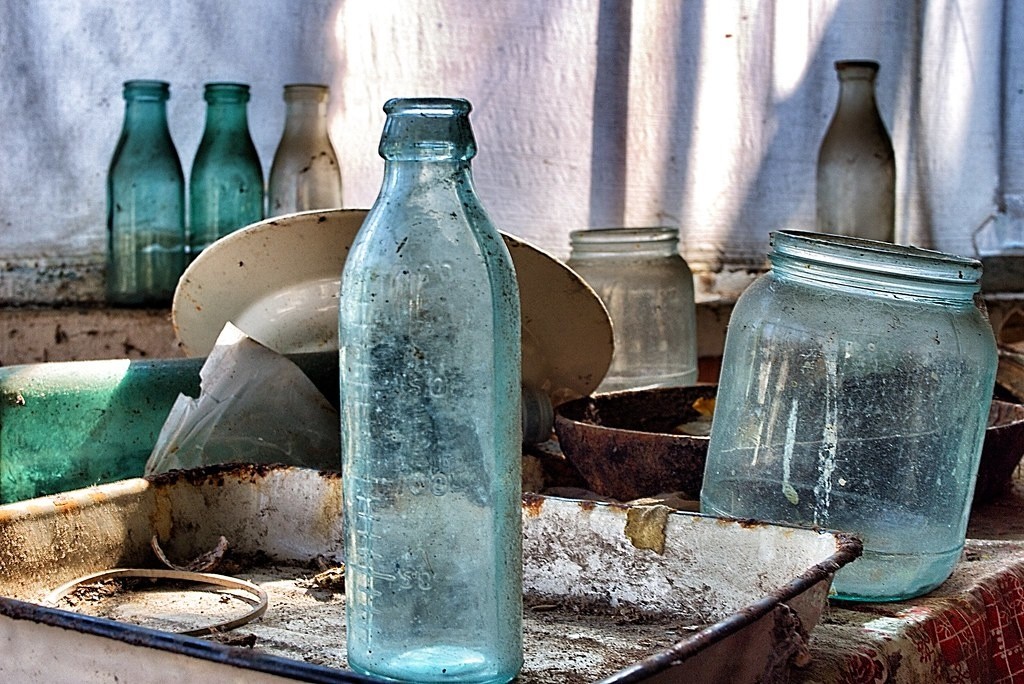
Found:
[338,96,528,684]
[563,226,699,402]
[814,59,895,252]
[700,229,998,606]
[180,82,265,293]
[103,80,187,310]
[268,82,346,226]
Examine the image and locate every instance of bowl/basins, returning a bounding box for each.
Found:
[172,206,613,404]
